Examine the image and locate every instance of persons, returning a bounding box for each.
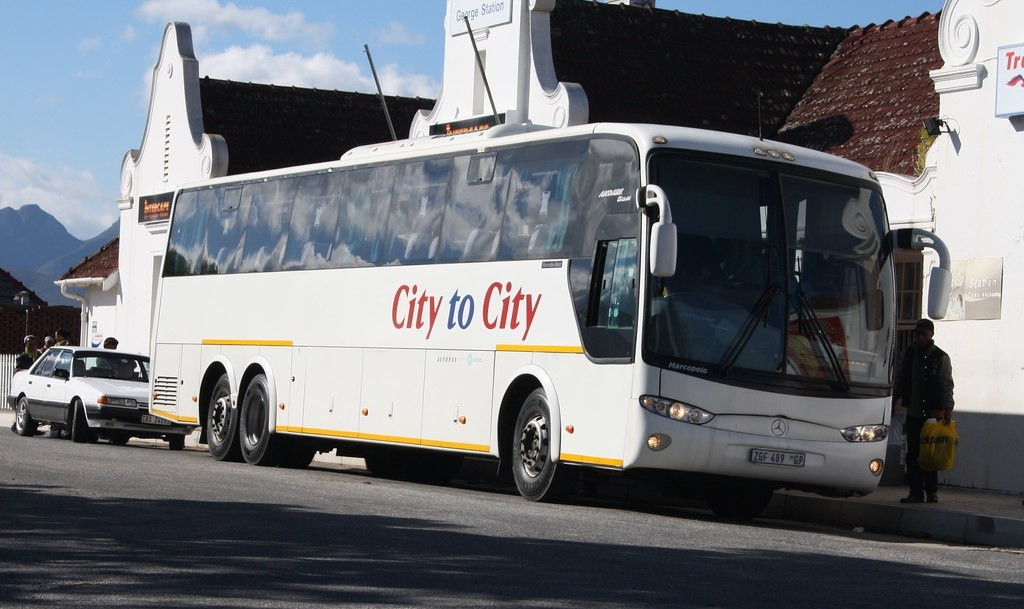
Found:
[55,328,72,346]
[37,336,55,355]
[894,315,953,503]
[11,334,47,437]
[96,336,118,368]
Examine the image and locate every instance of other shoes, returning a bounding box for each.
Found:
[900,493,925,503]
[925,494,939,503]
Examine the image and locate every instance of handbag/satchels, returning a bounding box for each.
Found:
[915,419,959,473]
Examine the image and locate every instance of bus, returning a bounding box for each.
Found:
[146,120,951,502]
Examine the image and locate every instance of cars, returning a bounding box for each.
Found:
[7,345,198,450]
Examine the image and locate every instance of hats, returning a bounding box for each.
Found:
[911,318,934,333]
